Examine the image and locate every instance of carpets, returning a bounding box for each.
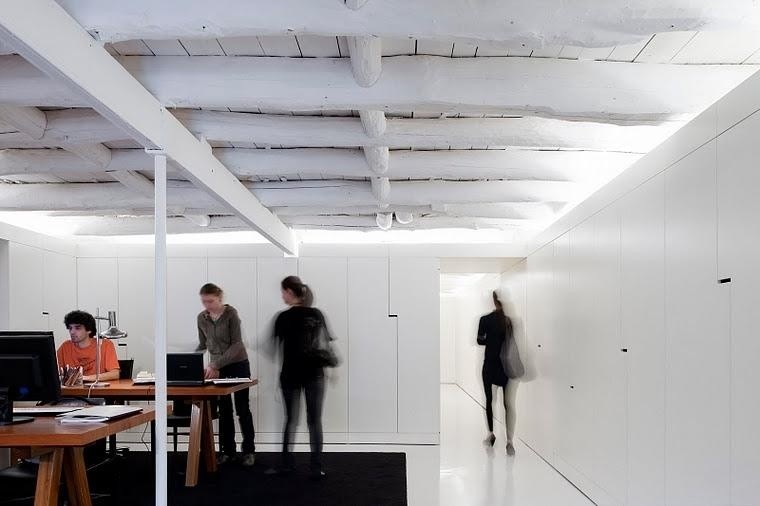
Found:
[0,451,407,506]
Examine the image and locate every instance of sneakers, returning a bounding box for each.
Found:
[242,453,257,467]
[214,450,238,467]
[272,457,297,472]
[309,465,329,481]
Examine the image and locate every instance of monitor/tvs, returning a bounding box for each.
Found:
[0,330,61,427]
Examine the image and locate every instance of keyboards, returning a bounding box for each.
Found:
[13,401,85,413]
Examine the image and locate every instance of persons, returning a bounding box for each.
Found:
[474,288,526,456]
[192,281,255,468]
[54,309,121,383]
[272,274,332,481]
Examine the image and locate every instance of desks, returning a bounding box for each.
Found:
[60,378,258,486]
[0,404,172,506]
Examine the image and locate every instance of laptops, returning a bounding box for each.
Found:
[166,351,213,385]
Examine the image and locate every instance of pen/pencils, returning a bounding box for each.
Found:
[63,365,82,386]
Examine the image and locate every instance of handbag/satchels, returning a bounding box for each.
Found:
[304,324,340,368]
